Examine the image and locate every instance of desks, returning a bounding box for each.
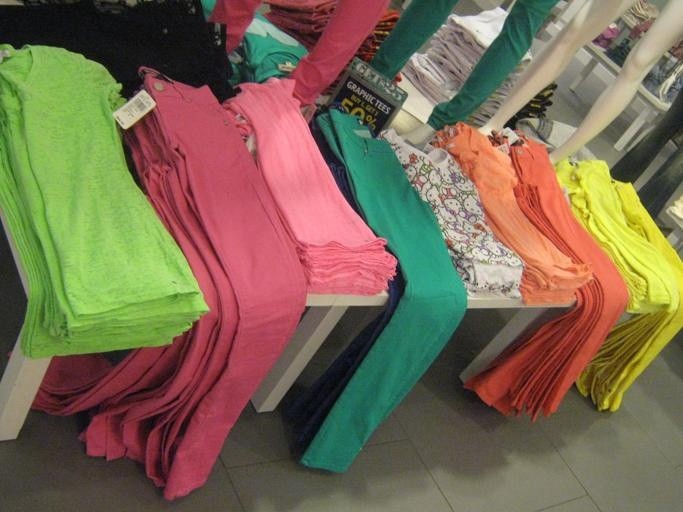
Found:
[0,206,577,441]
[559,16,678,151]
